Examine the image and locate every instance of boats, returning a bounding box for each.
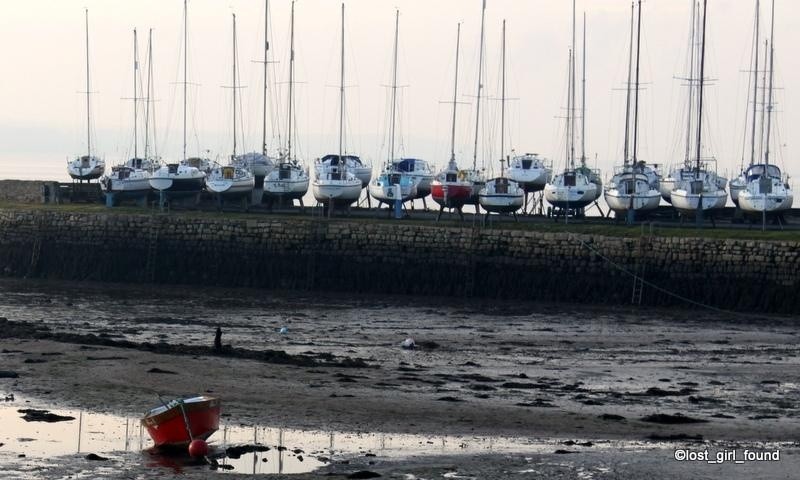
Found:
[139,390,221,459]
